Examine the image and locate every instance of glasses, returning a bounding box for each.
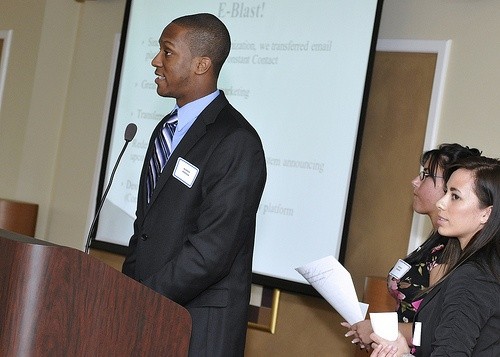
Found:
[420,171,444,181]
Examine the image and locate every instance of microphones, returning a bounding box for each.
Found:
[85,123,137,254]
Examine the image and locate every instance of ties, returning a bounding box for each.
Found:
[145,110,178,204]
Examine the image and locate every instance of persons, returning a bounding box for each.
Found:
[369,154,500,357]
[342,143,482,354]
[121,11,267,356]
